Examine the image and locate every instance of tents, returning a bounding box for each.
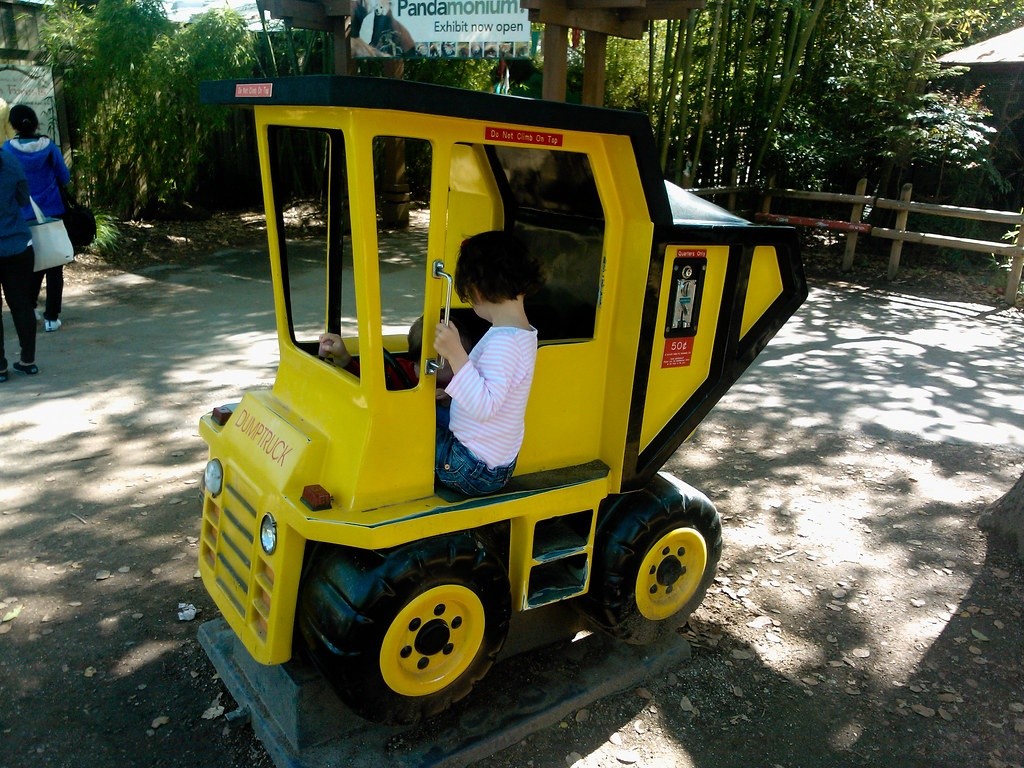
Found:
[934,26,1023,74]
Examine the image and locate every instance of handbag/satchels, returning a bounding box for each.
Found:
[25,195,75,273]
[57,179,96,246]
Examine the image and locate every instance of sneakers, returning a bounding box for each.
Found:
[12,362,38,376]
[44,318,62,332]
[0,371,8,382]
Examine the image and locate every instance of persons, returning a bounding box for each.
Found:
[434,230,544,497]
[0,105,71,331]
[319,315,453,390]
[0,148,38,383]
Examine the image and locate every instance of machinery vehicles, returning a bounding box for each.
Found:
[193,68,812,715]
